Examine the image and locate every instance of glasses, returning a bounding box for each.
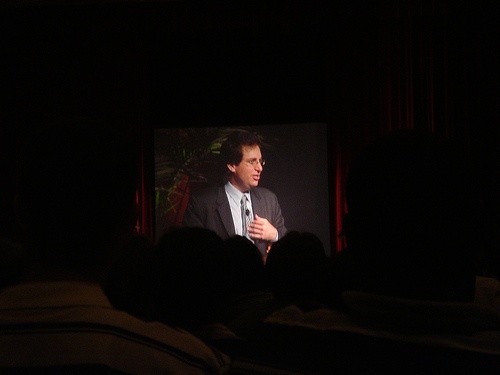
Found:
[243,159,265,167]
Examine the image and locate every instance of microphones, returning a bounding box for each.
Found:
[246,209,250,216]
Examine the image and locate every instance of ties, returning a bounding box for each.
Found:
[240,196,248,236]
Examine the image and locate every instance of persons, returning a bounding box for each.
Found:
[182,132,290,266]
[100,224,500,375]
[0,121,233,375]
